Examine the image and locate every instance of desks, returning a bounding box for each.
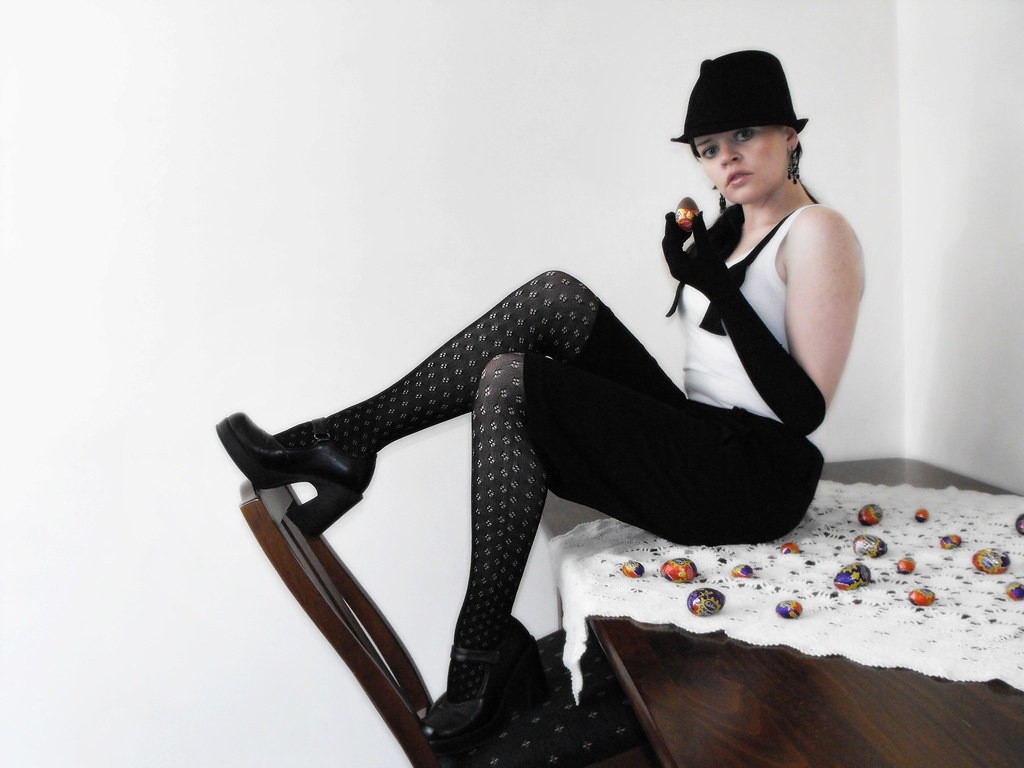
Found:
[545,455,1024,767]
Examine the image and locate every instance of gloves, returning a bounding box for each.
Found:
[662,211,826,437]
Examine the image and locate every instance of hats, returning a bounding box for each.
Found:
[671,50,810,143]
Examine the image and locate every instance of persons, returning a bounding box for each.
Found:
[217,51,866,754]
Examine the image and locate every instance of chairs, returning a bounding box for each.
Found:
[236,476,654,768]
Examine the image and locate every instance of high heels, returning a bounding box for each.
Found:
[421,615,548,757]
[217,412,378,540]
